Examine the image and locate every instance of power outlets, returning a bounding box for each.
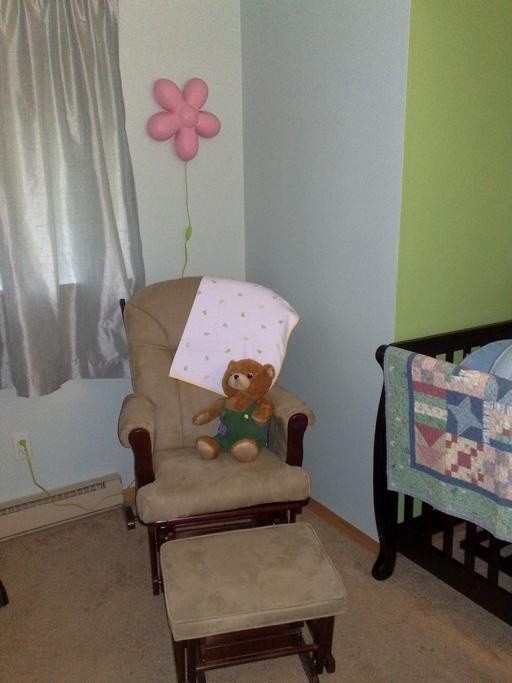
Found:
[11,431,32,461]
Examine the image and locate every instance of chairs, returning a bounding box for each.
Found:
[118,277,316,595]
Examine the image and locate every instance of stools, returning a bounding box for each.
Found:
[159,523,347,682]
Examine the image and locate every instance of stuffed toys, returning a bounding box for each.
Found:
[193,357,276,462]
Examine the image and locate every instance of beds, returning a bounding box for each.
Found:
[370,318,512,627]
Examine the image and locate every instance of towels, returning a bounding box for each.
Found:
[169,275,300,397]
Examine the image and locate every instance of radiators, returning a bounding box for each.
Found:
[0,472,125,543]
